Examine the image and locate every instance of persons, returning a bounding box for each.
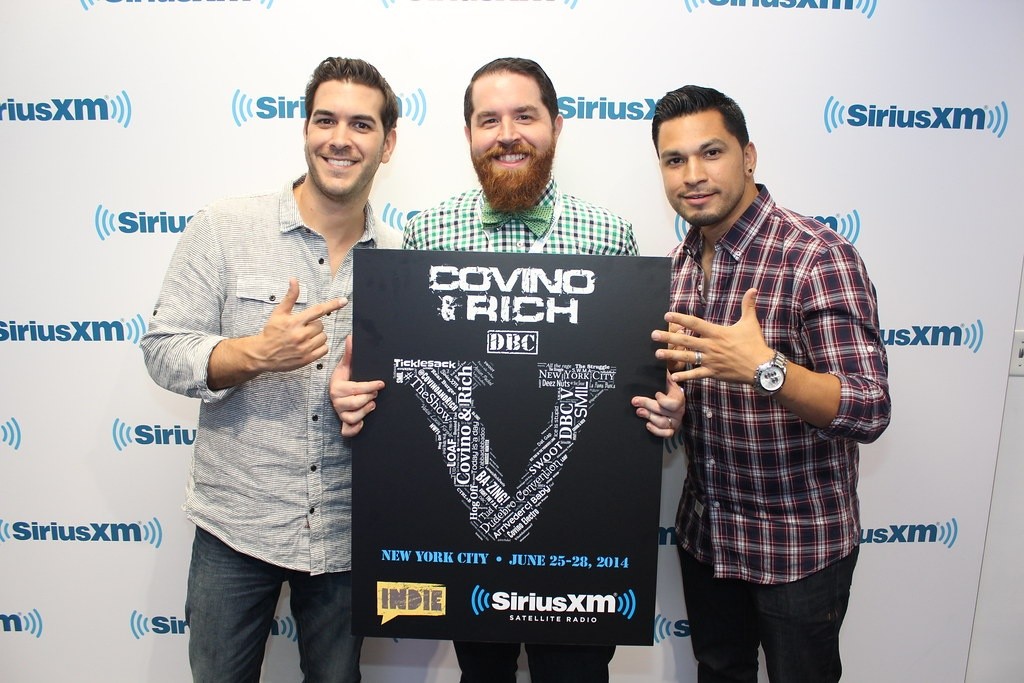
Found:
[142,57,401,683]
[327,57,687,683]
[651,84,890,682]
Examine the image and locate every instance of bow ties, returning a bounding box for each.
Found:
[481,202,554,238]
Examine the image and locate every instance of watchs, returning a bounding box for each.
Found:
[752,349,788,398]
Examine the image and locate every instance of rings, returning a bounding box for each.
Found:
[667,417,672,428]
[694,351,702,368]
